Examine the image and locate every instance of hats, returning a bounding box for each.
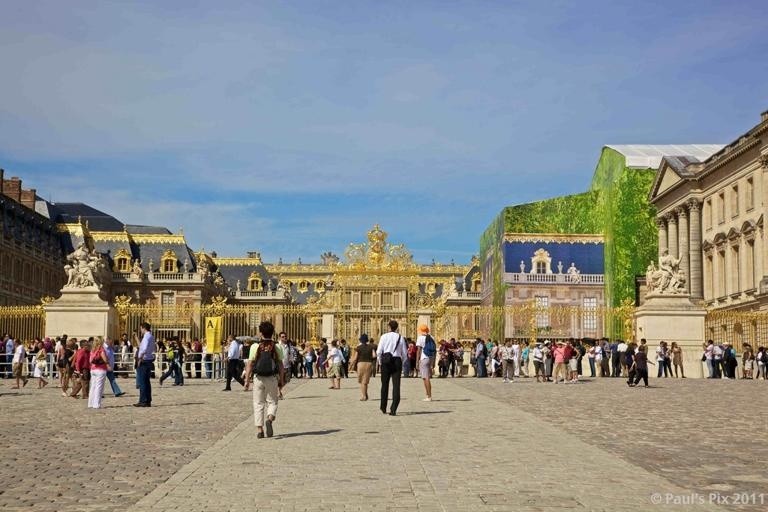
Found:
[418,323,429,333]
[358,334,369,341]
[533,339,566,349]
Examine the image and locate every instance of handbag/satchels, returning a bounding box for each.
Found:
[380,351,393,366]
[22,350,106,376]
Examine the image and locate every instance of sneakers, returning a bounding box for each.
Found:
[420,394,434,402]
[359,396,368,402]
[265,420,274,438]
[389,410,397,416]
[703,374,768,381]
[131,401,152,408]
[159,375,185,387]
[256,431,264,439]
[289,368,521,390]
[588,371,688,390]
[221,387,231,392]
[12,379,127,409]
[379,406,386,414]
[523,374,583,385]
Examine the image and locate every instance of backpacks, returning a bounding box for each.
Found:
[422,334,436,358]
[253,340,280,376]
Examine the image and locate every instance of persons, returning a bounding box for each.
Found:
[567,263,581,283]
[132,258,145,279]
[645,247,686,295]
[63,241,110,288]
